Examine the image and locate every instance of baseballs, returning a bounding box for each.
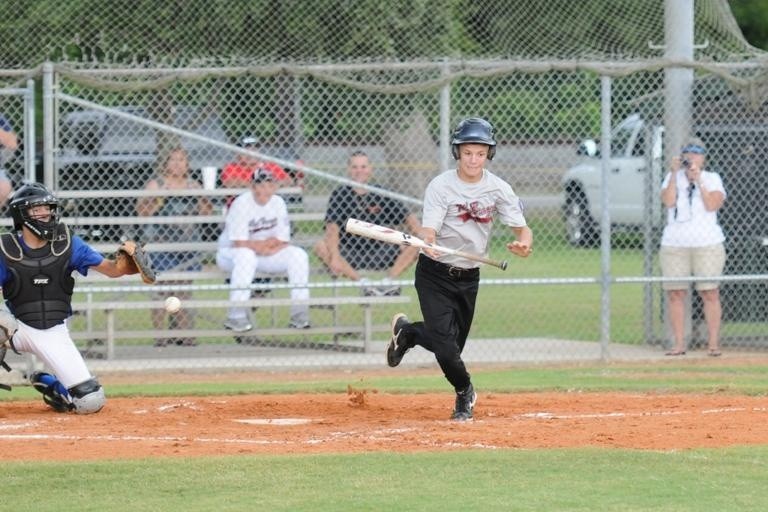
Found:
[165,297,180,312]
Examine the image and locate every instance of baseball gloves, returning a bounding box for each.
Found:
[114,241,157,284]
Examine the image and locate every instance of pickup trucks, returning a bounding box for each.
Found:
[7,105,306,244]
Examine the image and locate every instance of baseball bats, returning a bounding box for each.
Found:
[346,217,508,270]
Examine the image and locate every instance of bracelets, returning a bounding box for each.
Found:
[697,182,704,188]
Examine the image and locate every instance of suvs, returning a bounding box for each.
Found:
[560,114,674,253]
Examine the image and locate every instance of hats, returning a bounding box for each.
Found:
[682,144,704,154]
[237,136,262,148]
[252,169,272,183]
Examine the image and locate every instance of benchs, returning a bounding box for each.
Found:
[52,184,412,359]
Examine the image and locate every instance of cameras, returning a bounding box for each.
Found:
[681,158,691,168]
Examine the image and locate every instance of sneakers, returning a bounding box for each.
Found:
[224,317,250,332]
[451,386,477,423]
[289,315,312,328]
[386,313,409,366]
[31,372,68,412]
[359,277,401,294]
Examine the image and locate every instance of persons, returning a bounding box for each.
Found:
[658,138,730,359]
[1,180,144,415]
[133,145,210,350]
[221,162,308,333]
[320,140,421,296]
[0,117,19,204]
[217,131,289,208]
[387,115,533,423]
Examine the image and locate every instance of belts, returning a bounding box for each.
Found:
[419,254,479,279]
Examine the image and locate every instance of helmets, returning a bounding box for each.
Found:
[452,118,497,160]
[10,183,62,242]
